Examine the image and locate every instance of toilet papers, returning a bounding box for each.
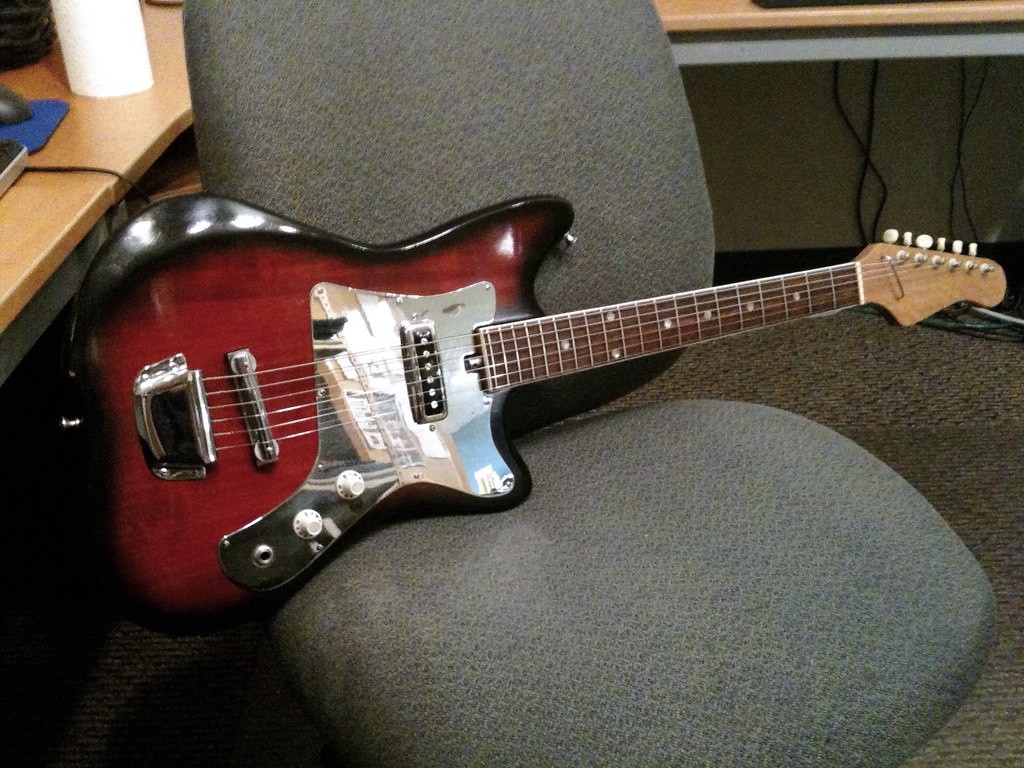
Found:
[42,1,157,98]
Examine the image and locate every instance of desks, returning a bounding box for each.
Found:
[0,1,1024,400]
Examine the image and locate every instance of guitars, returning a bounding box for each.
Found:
[68,191,1009,638]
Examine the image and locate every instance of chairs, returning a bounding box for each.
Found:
[183,0,1001,768]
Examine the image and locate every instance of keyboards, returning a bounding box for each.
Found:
[0,140,29,199]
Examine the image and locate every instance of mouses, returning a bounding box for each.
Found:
[0,84,34,126]
